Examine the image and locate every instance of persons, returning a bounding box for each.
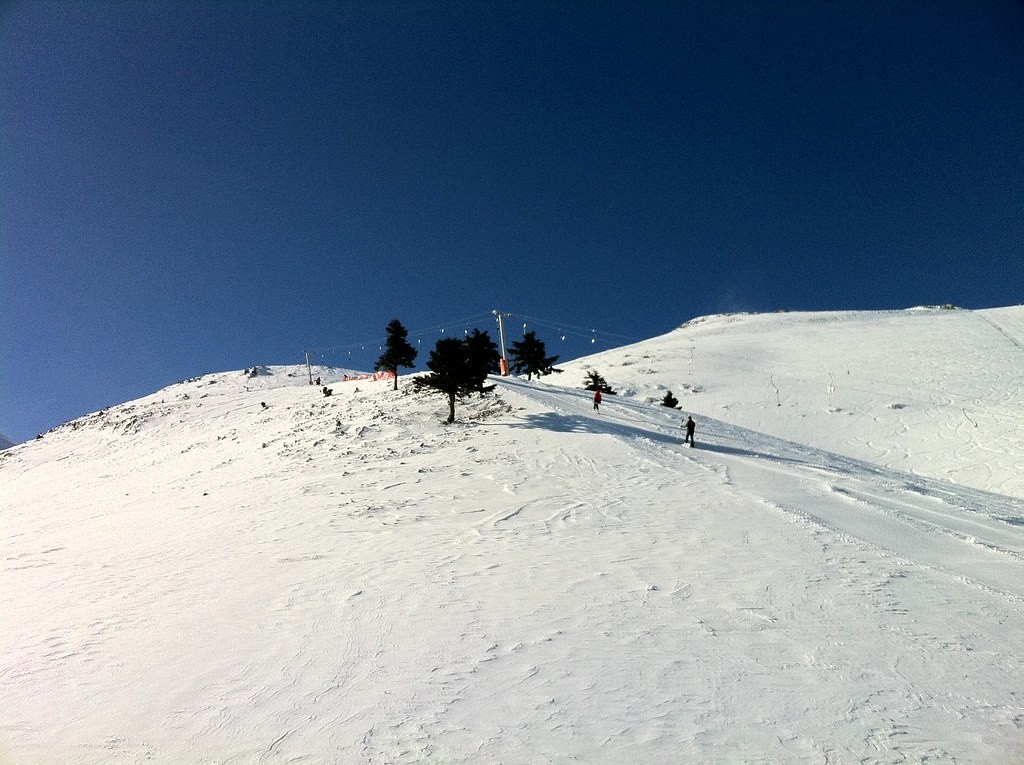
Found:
[316,377,321,385]
[680,416,696,447]
[593,390,601,414]
